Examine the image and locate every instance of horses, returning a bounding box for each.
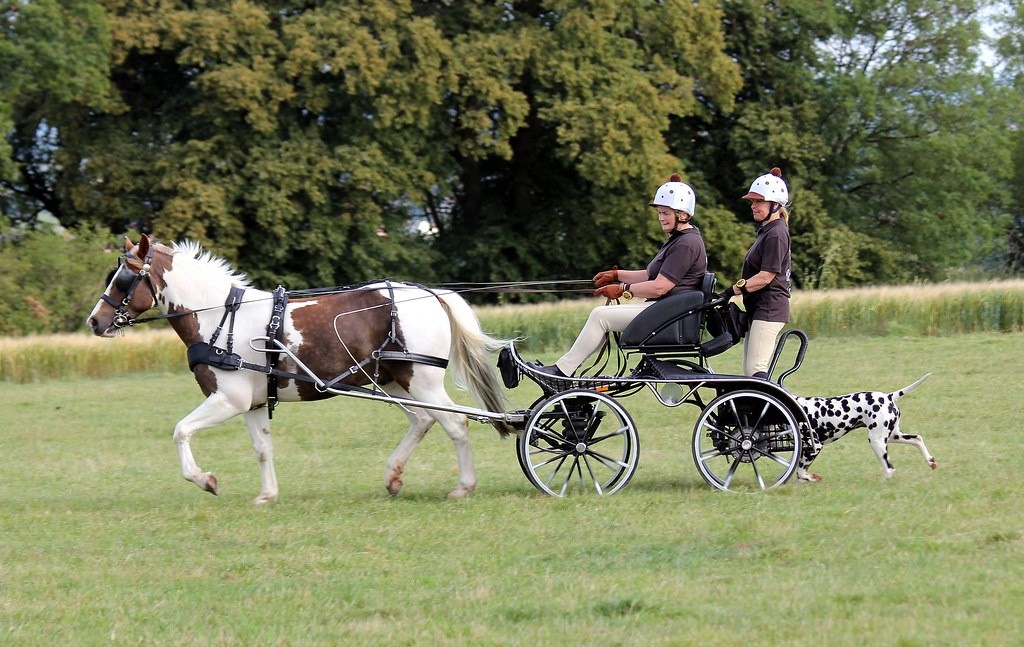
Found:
[84,233,531,507]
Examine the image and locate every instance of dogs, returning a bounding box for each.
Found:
[777,372,937,484]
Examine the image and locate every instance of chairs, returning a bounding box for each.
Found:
[618,271,717,351]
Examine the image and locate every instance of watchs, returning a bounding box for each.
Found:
[622,283,635,301]
[734,277,752,297]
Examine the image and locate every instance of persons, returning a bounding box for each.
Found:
[713,165,795,385]
[520,173,711,392]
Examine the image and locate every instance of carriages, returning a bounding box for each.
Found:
[87,233,811,496]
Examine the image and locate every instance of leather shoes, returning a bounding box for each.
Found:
[524,361,572,391]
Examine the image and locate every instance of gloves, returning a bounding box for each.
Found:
[593,283,623,299]
[592,266,617,287]
[720,287,735,307]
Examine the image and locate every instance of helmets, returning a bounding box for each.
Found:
[742,167,788,206]
[649,173,695,217]
